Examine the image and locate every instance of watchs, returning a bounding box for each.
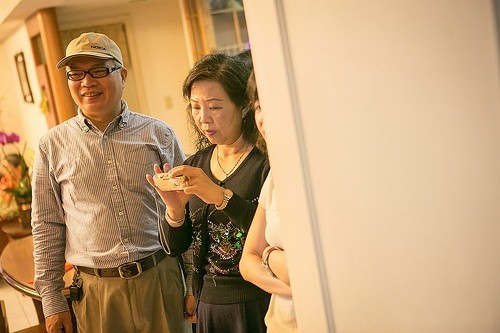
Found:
[215,189,233,210]
[261,246,284,279]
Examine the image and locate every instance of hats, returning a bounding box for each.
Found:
[56,30,123,69]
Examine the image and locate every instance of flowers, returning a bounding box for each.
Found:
[0,131,34,196]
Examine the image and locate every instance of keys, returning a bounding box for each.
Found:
[69,270,82,301]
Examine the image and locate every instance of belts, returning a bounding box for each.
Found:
[72,246,167,279]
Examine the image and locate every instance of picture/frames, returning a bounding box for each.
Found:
[14,52,33,104]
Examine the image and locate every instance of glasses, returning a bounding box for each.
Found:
[66,66,122,81]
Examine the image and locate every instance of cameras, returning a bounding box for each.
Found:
[153,173,187,192]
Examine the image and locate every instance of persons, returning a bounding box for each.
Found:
[1,155,32,206]
[239,69,298,333]
[144,52,272,333]
[32,31,195,333]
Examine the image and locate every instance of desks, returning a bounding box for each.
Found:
[0,235,77,333]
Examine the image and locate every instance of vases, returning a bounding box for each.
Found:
[14,196,32,230]
